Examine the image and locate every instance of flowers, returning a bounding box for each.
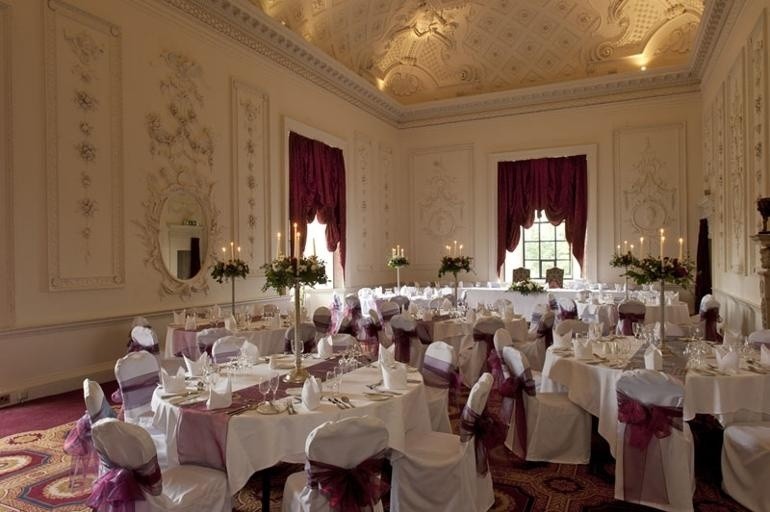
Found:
[438,256,473,278]
[206,255,249,283]
[259,255,329,296]
[609,252,699,290]
[387,256,410,268]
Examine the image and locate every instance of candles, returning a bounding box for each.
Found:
[445,240,463,257]
[392,244,404,258]
[616,229,684,262]
[275,223,302,260]
[222,241,241,263]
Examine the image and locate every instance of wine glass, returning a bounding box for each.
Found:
[259,377,269,402]
[334,366,345,394]
[326,372,337,394]
[202,356,253,378]
[270,372,280,401]
[588,322,755,372]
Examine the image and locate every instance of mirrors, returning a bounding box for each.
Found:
[129,167,224,305]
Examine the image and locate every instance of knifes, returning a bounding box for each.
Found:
[335,398,349,408]
[329,399,346,410]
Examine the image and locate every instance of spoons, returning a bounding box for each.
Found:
[341,396,355,408]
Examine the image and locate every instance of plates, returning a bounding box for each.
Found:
[169,396,209,406]
[256,405,287,414]
[365,394,391,400]
